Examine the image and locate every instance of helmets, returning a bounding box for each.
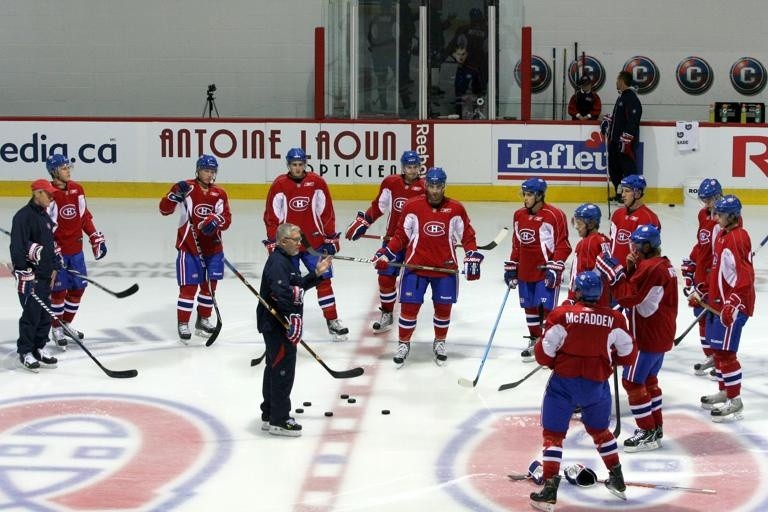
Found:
[620,174,646,191]
[627,224,660,249]
[521,177,546,192]
[697,178,722,198]
[711,194,742,218]
[573,203,601,226]
[573,271,603,302]
[194,148,447,190]
[45,154,71,176]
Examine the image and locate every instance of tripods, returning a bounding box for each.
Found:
[202,95,219,118]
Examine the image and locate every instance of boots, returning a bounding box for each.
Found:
[529,475,561,504]
[604,464,626,493]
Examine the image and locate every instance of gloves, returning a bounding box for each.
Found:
[196,213,224,236]
[373,245,398,270]
[463,250,484,280]
[681,259,696,277]
[88,231,107,260]
[269,285,304,306]
[11,268,35,298]
[167,180,193,203]
[719,292,745,328]
[594,251,626,286]
[24,239,43,265]
[54,246,67,270]
[504,260,519,289]
[345,210,371,241]
[285,314,302,346]
[688,282,708,308]
[544,260,564,289]
[262,239,275,256]
[682,277,693,296]
[323,233,342,256]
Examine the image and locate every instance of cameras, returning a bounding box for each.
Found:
[206,84,216,95]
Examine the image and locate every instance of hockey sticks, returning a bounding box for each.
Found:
[506,473,717,494]
[537,301,548,328]
[674,234,767,347]
[251,347,269,365]
[1,228,139,297]
[611,346,621,439]
[182,195,222,346]
[605,128,612,222]
[499,364,543,391]
[222,258,364,378]
[3,262,138,378]
[301,231,466,274]
[362,226,508,251]
[458,280,512,388]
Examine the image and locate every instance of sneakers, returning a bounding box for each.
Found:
[633,425,662,438]
[61,321,84,340]
[51,326,67,347]
[432,338,446,361]
[372,311,393,330]
[30,347,57,364]
[269,416,301,431]
[606,193,620,201]
[261,414,294,422]
[520,337,540,357]
[326,318,348,335]
[709,368,716,376]
[616,199,624,203]
[195,315,215,333]
[177,321,191,339]
[693,355,714,371]
[699,390,727,405]
[393,341,409,364]
[18,352,39,370]
[623,430,656,447]
[710,398,742,416]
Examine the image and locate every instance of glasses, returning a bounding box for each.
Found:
[283,236,301,242]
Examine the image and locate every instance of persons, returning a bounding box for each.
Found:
[346,150,432,330]
[264,147,349,335]
[256,223,333,431]
[159,154,232,340]
[37,153,107,346]
[606,71,643,203]
[9,178,61,369]
[441,42,482,117]
[372,167,483,364]
[568,74,601,120]
[447,7,491,120]
[503,172,756,512]
[366,0,417,110]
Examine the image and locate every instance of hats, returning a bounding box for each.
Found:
[30,179,60,194]
[580,75,591,84]
